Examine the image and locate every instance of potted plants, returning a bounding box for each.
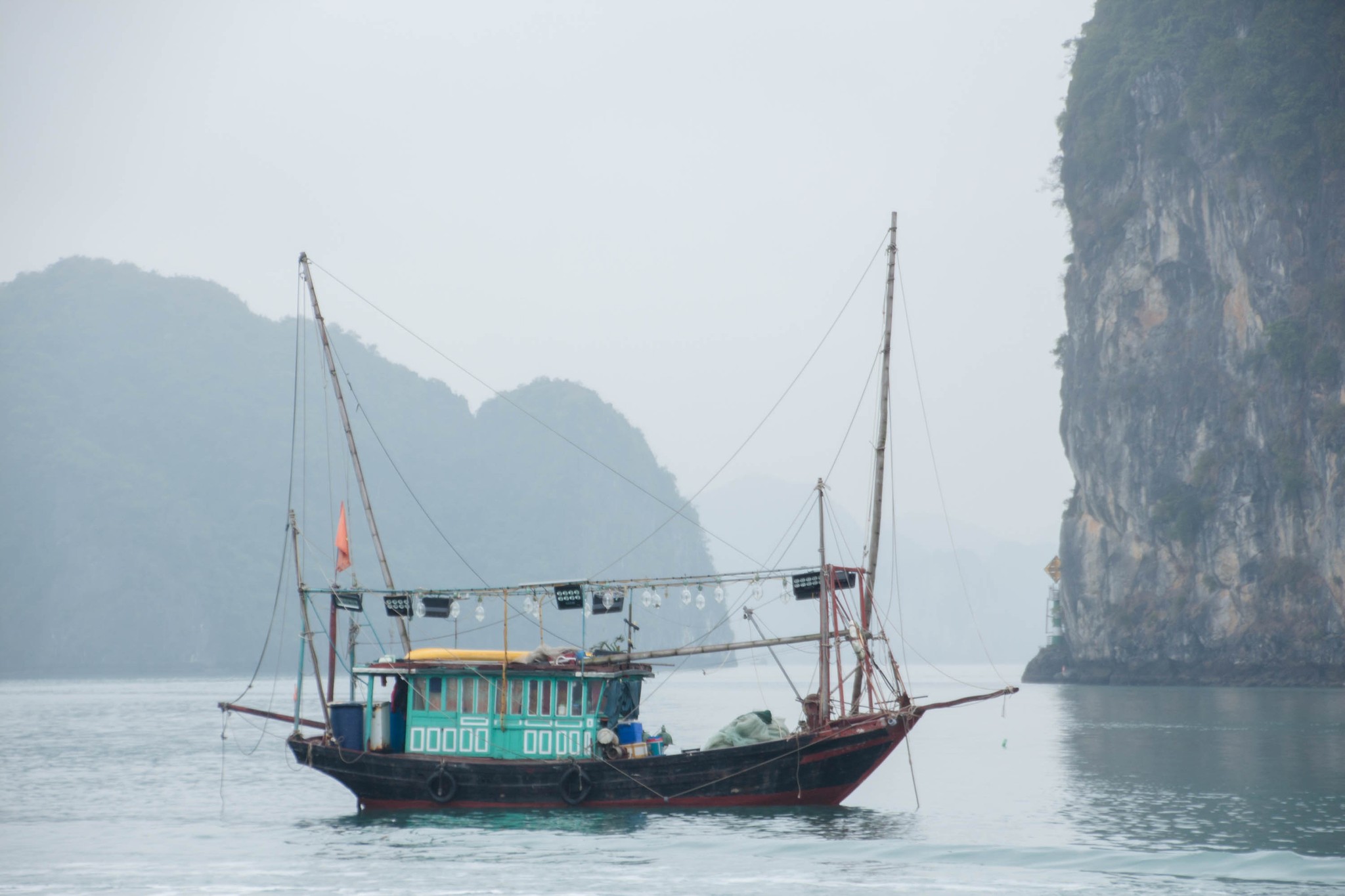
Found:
[585,635,634,664]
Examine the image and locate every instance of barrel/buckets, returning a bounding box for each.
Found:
[327,700,403,749]
[606,747,622,760]
[575,650,585,661]
[646,736,665,757]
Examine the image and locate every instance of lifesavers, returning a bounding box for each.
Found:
[425,770,457,804]
[558,767,593,806]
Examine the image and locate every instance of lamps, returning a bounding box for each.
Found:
[554,585,583,610]
[384,596,414,617]
[334,593,363,612]
[835,571,856,590]
[592,592,624,614]
[792,572,820,600]
[422,597,454,618]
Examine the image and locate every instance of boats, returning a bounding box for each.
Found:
[217,210,1021,807]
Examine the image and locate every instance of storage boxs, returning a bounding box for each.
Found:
[326,701,390,751]
[617,720,648,759]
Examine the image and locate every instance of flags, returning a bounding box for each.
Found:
[334,500,351,573]
